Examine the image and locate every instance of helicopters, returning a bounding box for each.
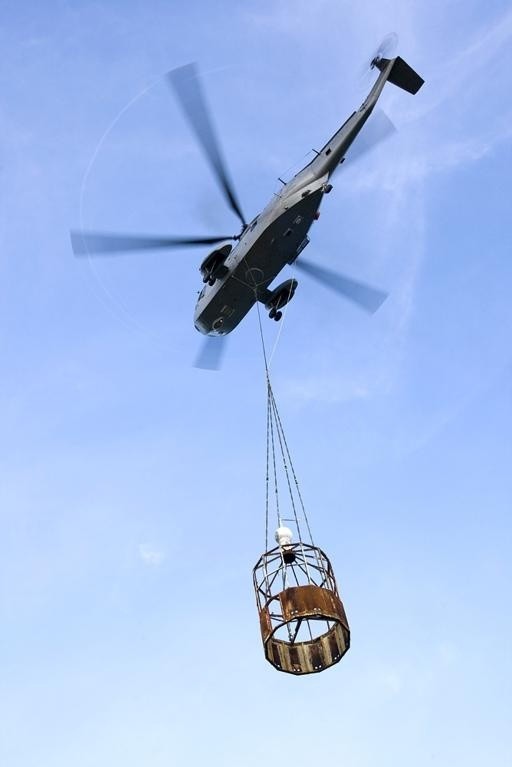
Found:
[67,29,429,383]
